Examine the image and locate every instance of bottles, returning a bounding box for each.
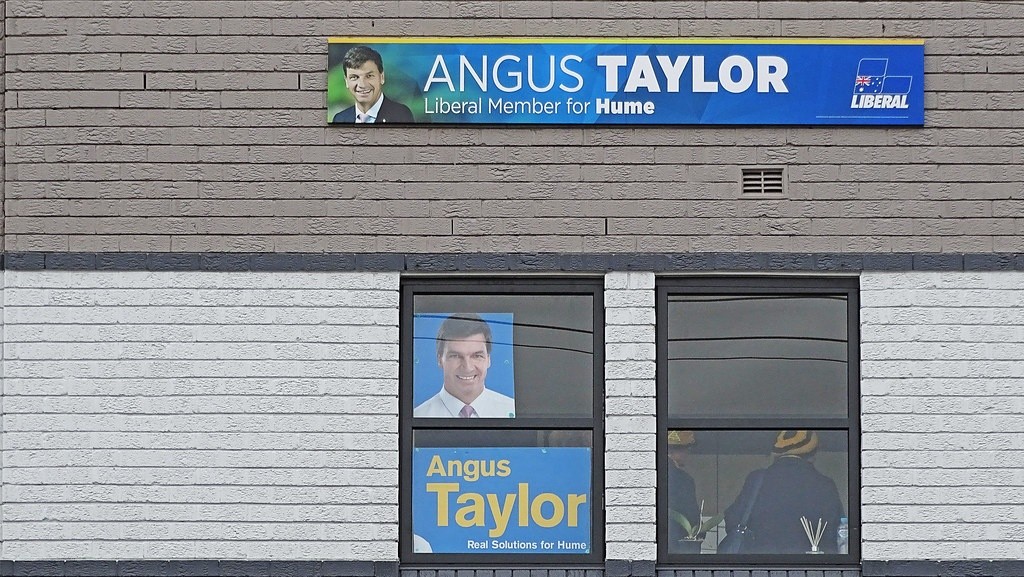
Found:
[837,517,848,555]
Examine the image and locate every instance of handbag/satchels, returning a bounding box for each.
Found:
[718,524,752,554]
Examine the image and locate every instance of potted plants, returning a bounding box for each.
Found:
[671,506,725,556]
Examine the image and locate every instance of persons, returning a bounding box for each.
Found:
[664,429,702,556]
[414,312,515,418]
[724,429,846,555]
[332,46,416,123]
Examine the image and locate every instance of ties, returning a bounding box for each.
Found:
[359,114,369,123]
[460,405,473,417]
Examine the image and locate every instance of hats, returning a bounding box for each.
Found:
[772,428,818,458]
[668,431,696,449]
